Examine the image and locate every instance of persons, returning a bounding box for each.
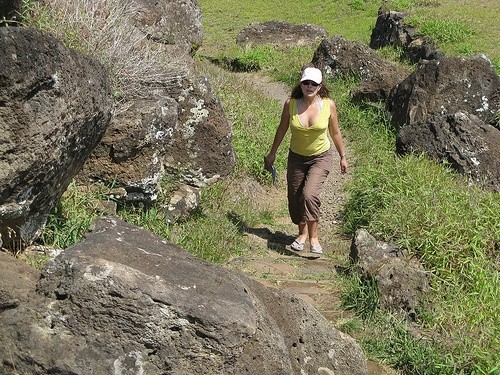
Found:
[265,66,348,254]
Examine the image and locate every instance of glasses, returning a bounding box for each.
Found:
[302,81,320,86]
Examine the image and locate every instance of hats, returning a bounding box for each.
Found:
[300,67,323,84]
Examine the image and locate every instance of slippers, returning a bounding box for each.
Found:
[290,237,304,251]
[309,245,323,254]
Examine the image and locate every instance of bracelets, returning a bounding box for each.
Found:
[339,153,346,157]
